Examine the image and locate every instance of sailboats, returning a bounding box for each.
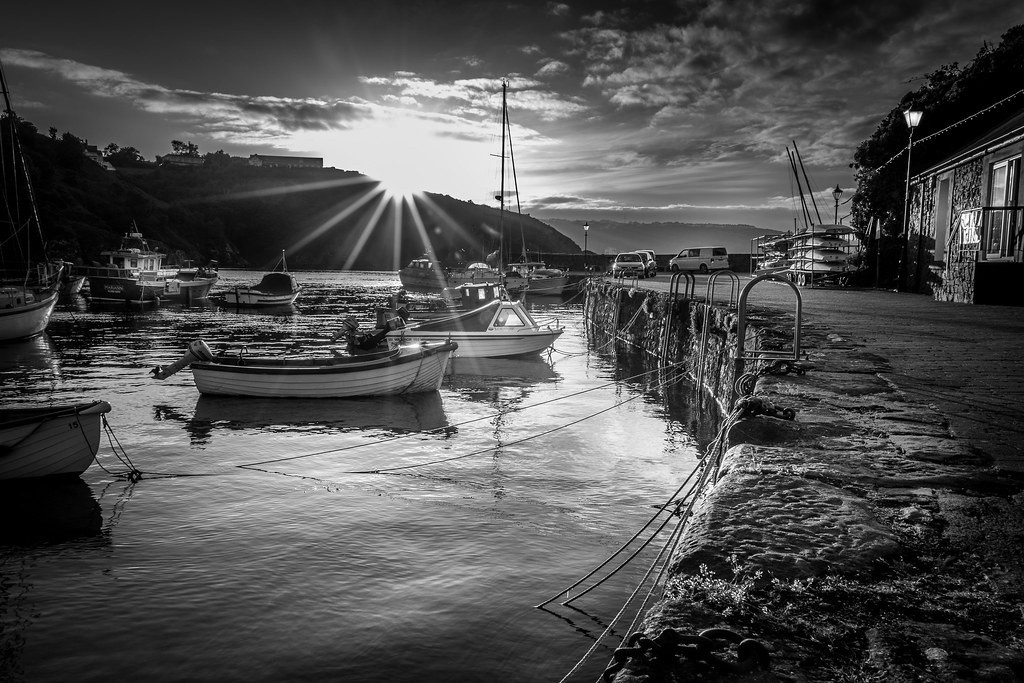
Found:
[0,60,64,339]
[398,82,569,294]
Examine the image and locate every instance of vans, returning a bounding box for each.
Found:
[669,247,729,274]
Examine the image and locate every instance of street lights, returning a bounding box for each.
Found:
[892,97,924,294]
[832,184,843,224]
[582,222,589,261]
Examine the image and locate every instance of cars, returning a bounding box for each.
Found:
[585,265,600,273]
[638,250,656,260]
[613,253,645,279]
[638,252,658,279]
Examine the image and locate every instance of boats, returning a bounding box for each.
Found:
[87,233,219,303]
[149,316,458,397]
[31,261,85,296]
[439,355,564,389]
[207,273,302,308]
[374,283,509,328]
[0,400,113,479]
[330,299,565,359]
[187,390,449,432]
